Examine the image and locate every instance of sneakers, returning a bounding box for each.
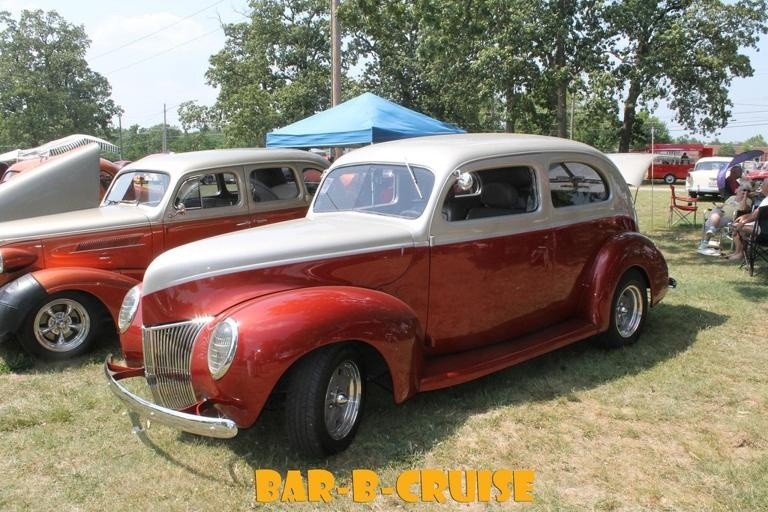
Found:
[695,229,723,257]
[723,252,745,261]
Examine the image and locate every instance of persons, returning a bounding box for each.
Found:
[696,166,768,260]
[680,153,690,165]
[302,155,334,182]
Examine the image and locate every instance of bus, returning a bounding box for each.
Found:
[634,143,714,185]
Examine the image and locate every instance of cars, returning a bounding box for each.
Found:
[0,150,161,210]
[101,129,680,463]
[0,142,333,365]
[685,155,742,199]
[742,161,768,184]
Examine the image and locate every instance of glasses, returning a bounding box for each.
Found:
[740,188,752,193]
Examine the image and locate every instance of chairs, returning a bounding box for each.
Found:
[728,206,767,280]
[700,197,755,251]
[461,181,524,217]
[667,180,698,230]
[411,177,461,220]
